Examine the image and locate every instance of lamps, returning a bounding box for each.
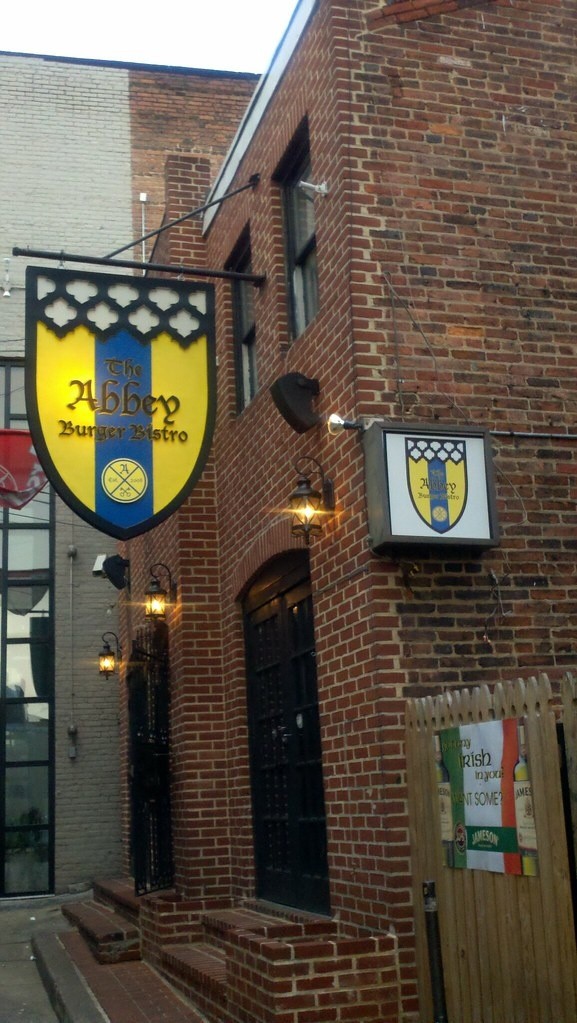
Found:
[102,554,128,589]
[289,456,334,549]
[98,632,122,680]
[145,562,176,626]
[270,372,319,434]
[327,414,362,435]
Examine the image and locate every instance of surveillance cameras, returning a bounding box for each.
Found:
[92,553,107,577]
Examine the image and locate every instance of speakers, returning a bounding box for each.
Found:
[102,554,127,589]
[269,371,320,433]
